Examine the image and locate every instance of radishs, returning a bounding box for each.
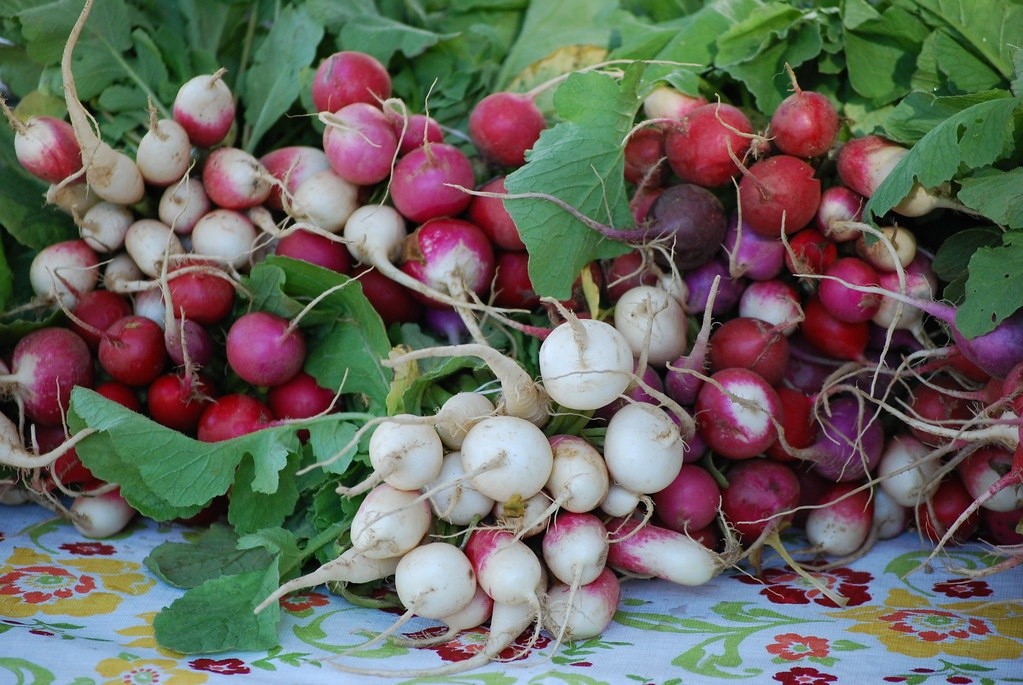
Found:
[60,0,688,679]
